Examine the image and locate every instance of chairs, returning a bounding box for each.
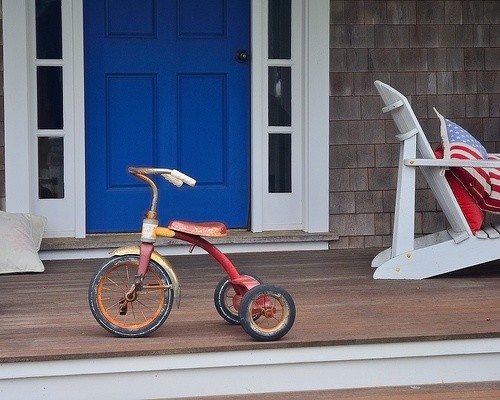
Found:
[370,80,500,279]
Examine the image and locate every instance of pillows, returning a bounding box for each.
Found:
[432,144,486,236]
[0,209,48,274]
[431,105,500,215]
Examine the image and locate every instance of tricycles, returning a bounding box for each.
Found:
[87,166,296,341]
